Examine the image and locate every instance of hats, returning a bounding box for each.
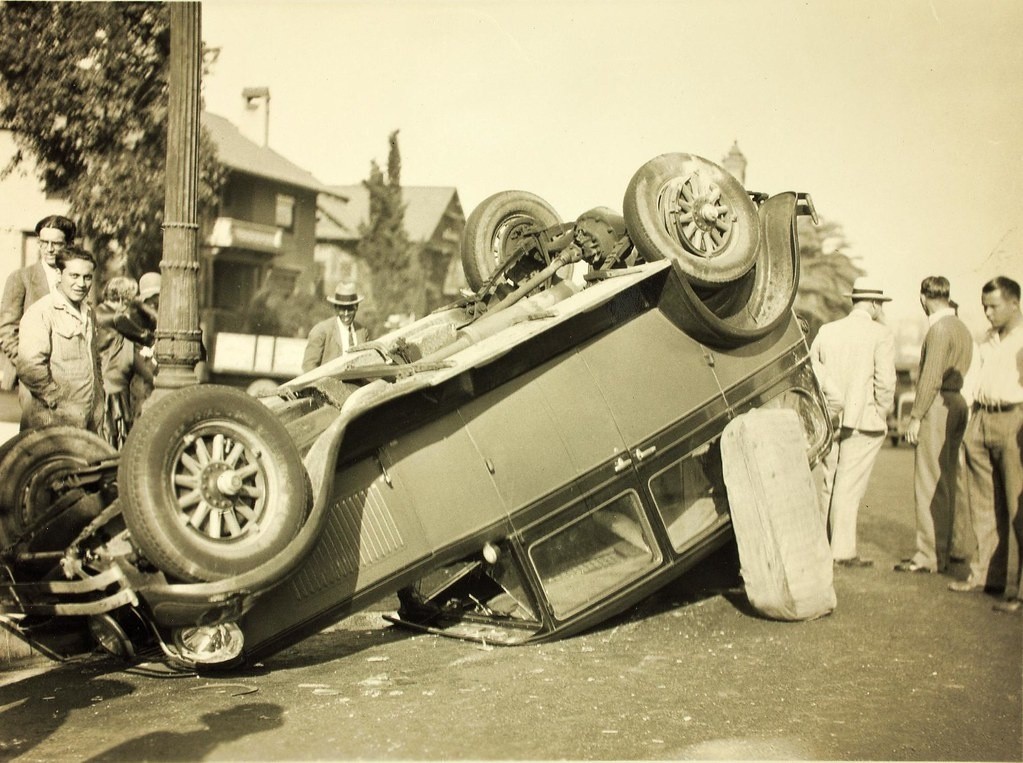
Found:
[327,282,364,305]
[844,276,892,302]
[134,272,161,303]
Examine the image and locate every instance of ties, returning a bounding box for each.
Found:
[349,326,354,347]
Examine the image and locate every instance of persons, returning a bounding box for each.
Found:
[809,276,897,568]
[947,276,1023,614]
[0,214,162,454]
[302,281,371,375]
[894,276,974,573]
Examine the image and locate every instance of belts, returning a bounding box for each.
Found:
[941,388,960,392]
[974,402,1015,412]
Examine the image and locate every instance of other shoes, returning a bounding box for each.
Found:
[832,556,875,567]
[894,557,933,573]
[946,579,999,592]
[994,598,1022,612]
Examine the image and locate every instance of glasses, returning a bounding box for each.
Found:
[38,239,65,250]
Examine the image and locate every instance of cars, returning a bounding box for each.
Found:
[5,143,893,681]
